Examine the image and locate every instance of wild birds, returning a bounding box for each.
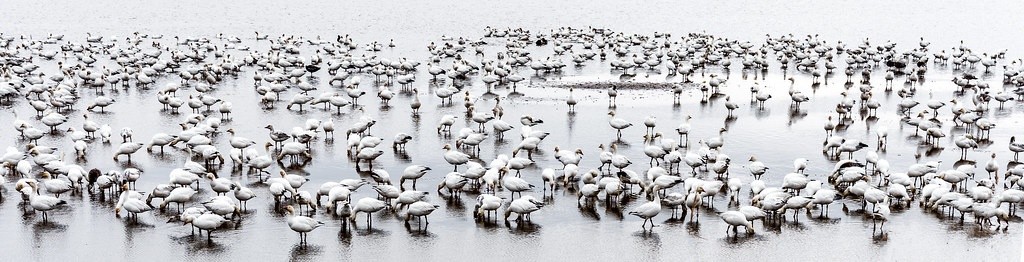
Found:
[0,25,1024,257]
